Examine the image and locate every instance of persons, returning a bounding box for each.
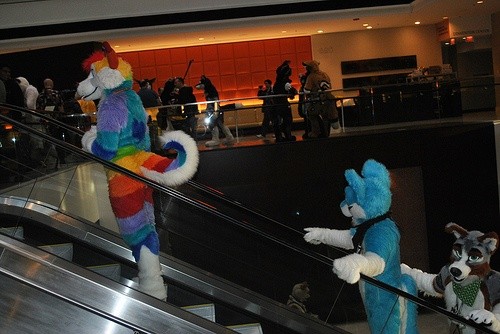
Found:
[258,78,279,137]
[173,76,197,140]
[35,78,66,165]
[158,86,164,103]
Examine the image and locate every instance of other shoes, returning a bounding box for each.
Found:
[60,157,66,164]
[256,133,264,138]
[40,160,48,168]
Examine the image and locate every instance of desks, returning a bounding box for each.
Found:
[234,90,360,144]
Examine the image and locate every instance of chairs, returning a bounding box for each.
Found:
[196,104,304,135]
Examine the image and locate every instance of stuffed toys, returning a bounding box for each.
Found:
[287,280,320,320]
[76,40,200,300]
[400,222,500,334]
[0,61,341,165]
[303,159,418,334]
